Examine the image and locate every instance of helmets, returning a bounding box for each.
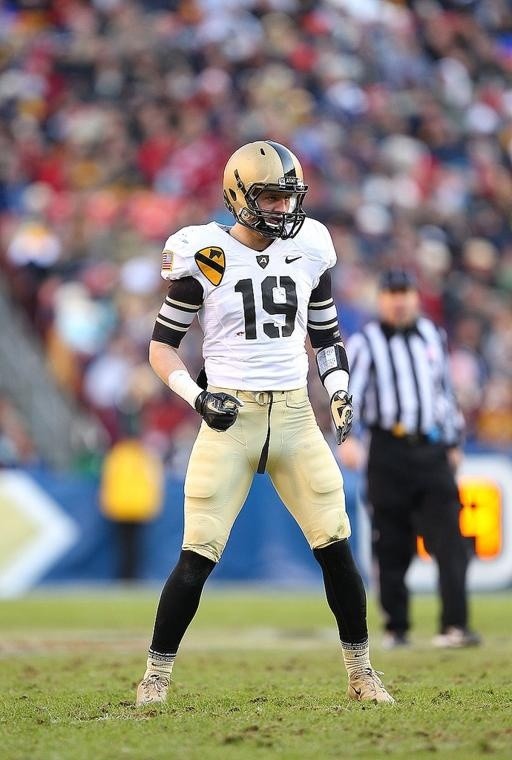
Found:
[221,139,306,223]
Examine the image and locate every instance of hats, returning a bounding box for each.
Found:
[373,267,419,292]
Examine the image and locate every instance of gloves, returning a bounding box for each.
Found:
[328,390,356,447]
[194,390,244,432]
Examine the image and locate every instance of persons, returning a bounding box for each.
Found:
[338,273,482,651]
[2,2,511,583]
[129,135,401,710]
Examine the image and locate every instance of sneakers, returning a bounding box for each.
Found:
[430,623,482,650]
[347,668,396,703]
[135,673,170,704]
[381,629,413,649]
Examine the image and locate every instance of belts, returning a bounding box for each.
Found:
[208,387,285,406]
